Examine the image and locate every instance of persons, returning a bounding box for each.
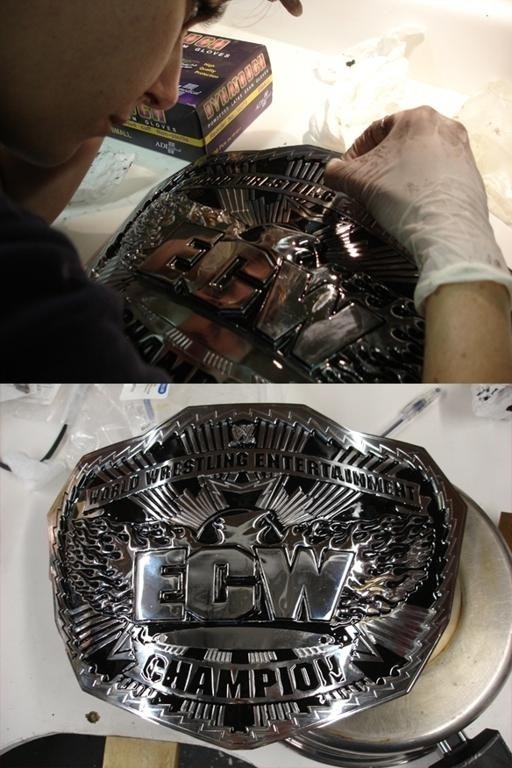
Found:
[2,0,512,385]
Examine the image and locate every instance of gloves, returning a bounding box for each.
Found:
[325,106,511,318]
